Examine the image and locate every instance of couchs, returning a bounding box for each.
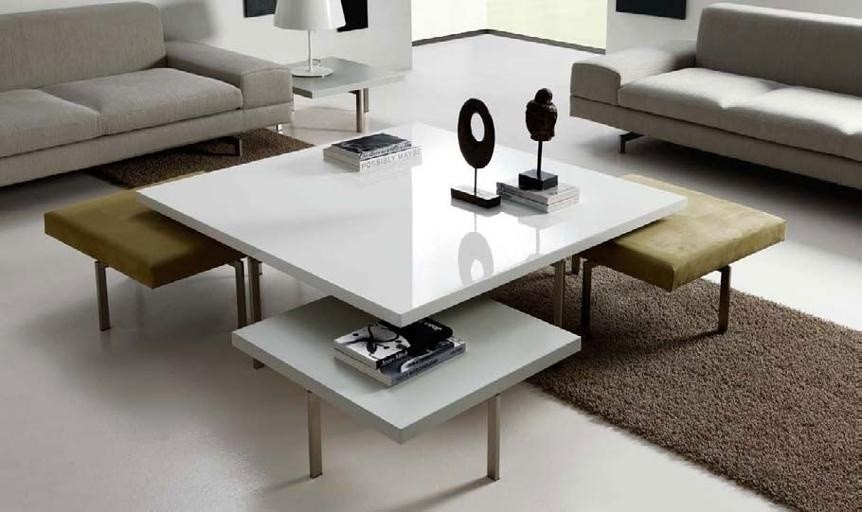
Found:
[0,0,293,187]
[570,4,862,190]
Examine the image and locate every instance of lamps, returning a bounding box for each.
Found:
[272,0,347,77]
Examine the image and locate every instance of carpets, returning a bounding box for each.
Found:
[88,127,862,512]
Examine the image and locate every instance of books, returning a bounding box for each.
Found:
[330,133,412,161]
[334,336,466,386]
[375,340,454,374]
[497,178,580,205]
[323,142,423,172]
[333,317,452,370]
[496,190,580,214]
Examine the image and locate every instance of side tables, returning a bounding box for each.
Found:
[275,55,405,132]
